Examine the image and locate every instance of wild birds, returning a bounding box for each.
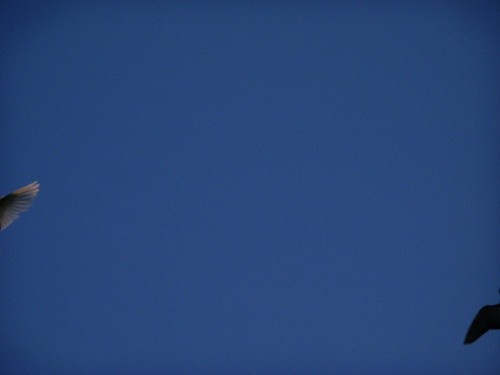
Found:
[464,288,500,345]
[0,180,40,233]
